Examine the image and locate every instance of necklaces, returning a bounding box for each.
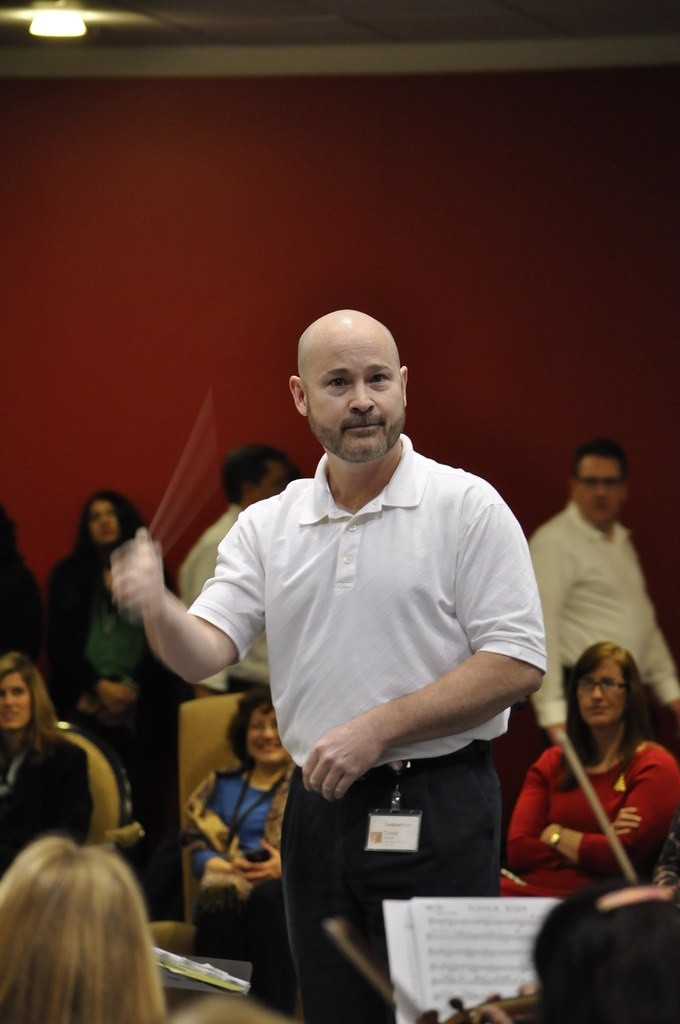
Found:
[222,764,286,853]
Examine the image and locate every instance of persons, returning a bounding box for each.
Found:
[0,439,680,1024]
[109,308,548,1024]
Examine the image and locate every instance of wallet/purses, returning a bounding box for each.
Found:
[76,691,136,729]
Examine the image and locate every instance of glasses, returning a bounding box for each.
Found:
[579,675,628,691]
[579,477,625,489]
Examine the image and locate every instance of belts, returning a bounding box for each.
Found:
[354,757,448,788]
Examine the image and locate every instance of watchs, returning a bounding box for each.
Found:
[550,826,564,850]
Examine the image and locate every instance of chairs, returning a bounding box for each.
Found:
[52,720,131,850]
[179,691,245,924]
